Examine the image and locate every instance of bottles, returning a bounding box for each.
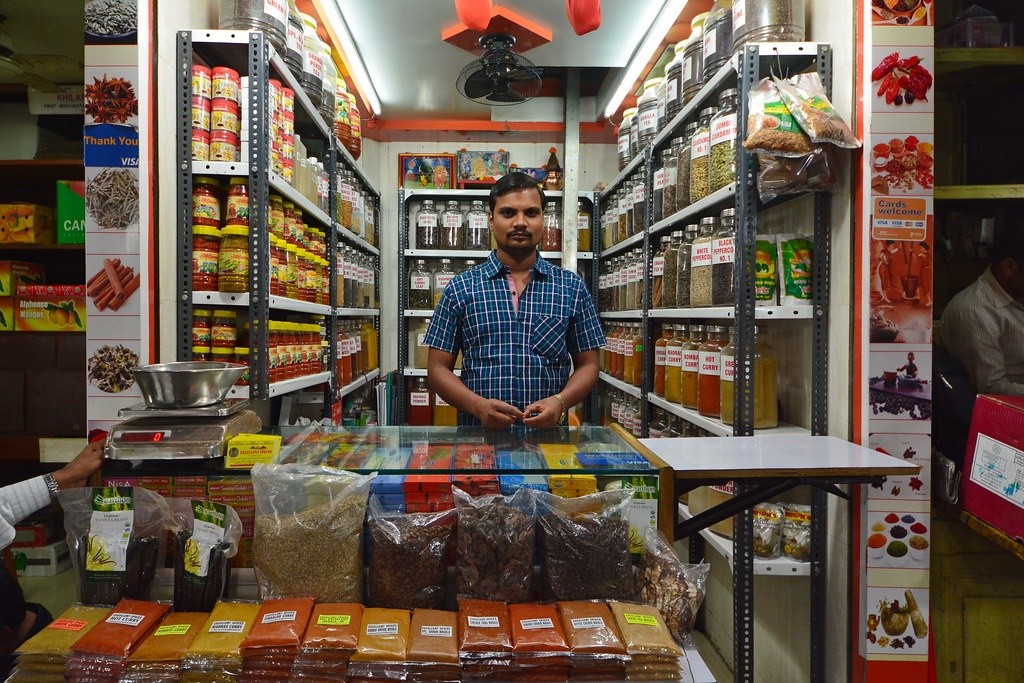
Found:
[185,1,818,494]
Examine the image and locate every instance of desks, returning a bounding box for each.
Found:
[98,428,919,570]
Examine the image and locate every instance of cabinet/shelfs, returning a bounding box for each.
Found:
[395,186,595,428]
[592,41,829,683]
[175,26,384,426]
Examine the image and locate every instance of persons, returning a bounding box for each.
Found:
[930,219,1024,463]
[0,438,108,552]
[419,172,607,434]
[0,559,57,683]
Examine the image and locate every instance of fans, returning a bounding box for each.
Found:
[455,32,540,107]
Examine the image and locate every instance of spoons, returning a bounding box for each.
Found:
[872,15,911,26]
[923,0,933,26]
[907,7,927,26]
[870,5,894,20]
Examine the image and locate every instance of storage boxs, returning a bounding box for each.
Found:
[9,538,73,578]
[9,524,56,547]
[0,204,55,244]
[0,295,14,331]
[15,286,87,332]
[55,180,86,244]
[0,260,49,296]
[960,392,1023,549]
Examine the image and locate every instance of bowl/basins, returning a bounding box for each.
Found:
[131,362,248,409]
[872,135,933,174]
[867,512,930,568]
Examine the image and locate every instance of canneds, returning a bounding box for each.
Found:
[184,0,384,386]
[396,0,807,439]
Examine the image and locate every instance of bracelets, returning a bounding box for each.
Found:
[44,472,62,500]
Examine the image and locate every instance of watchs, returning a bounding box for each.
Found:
[552,394,567,426]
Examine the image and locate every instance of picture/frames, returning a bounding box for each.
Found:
[457,148,510,180]
[398,153,455,190]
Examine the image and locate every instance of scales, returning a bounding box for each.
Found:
[102,398,262,461]
